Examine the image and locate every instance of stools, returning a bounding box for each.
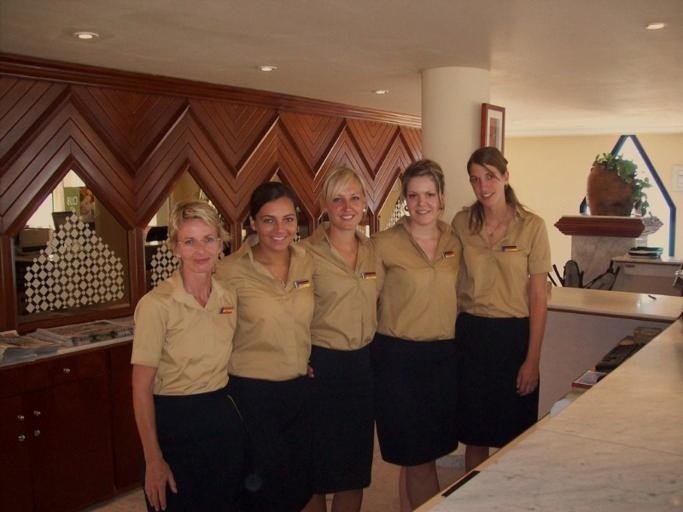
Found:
[19,228,49,251]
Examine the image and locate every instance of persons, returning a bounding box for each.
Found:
[368,159,458,512]
[212,181,317,512]
[130,199,245,512]
[296,165,387,512]
[451,147,553,474]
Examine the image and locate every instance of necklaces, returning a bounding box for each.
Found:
[484,207,510,240]
[262,257,288,288]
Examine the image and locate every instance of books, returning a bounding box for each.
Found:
[627,244,664,259]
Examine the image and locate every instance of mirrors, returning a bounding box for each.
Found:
[13,165,130,318]
[319,207,370,237]
[380,179,411,234]
[241,174,308,245]
[144,170,234,292]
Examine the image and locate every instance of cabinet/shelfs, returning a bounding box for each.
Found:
[1,343,117,512]
[117,336,146,501]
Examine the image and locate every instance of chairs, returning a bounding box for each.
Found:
[50,210,80,250]
[548,257,620,290]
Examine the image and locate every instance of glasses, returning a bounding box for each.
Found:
[174,234,222,251]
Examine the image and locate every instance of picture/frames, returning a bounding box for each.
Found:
[480,103,505,159]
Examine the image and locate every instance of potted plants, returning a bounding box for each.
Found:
[586,153,653,227]
